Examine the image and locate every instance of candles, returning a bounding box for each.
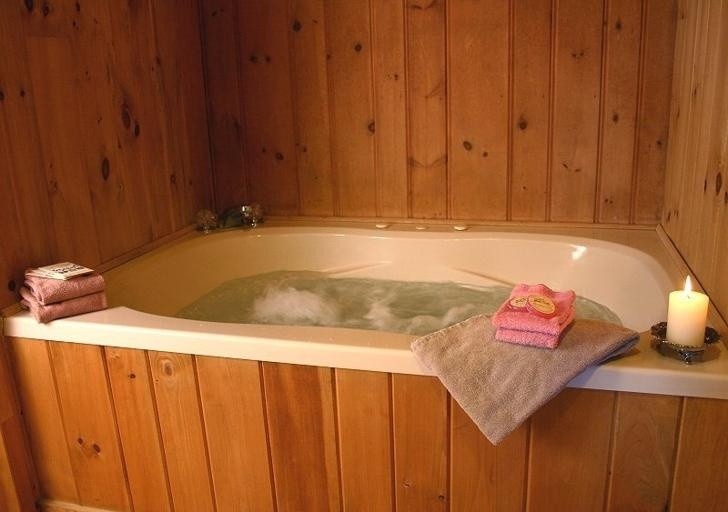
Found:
[664,275,714,346]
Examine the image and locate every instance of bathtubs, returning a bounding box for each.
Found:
[3,213,728,401]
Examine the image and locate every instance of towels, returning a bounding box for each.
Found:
[495,283,577,336]
[491,326,571,348]
[12,282,108,325]
[18,265,104,305]
[410,306,641,447]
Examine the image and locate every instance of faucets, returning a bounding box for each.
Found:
[218,203,261,229]
